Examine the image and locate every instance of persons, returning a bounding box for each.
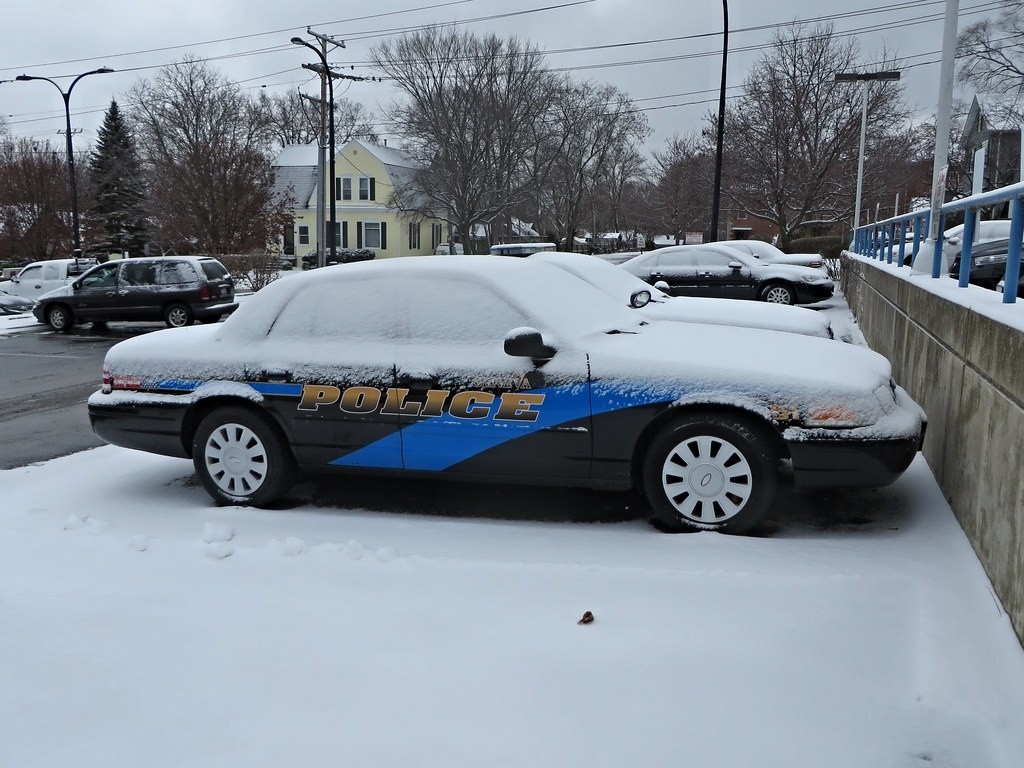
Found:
[97,252,110,263]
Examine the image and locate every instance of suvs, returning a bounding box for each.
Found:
[34,253,240,335]
[1,256,109,312]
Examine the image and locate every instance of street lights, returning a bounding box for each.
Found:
[835,70,901,248]
[16,66,114,258]
[291,37,338,267]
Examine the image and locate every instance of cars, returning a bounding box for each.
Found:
[536,253,834,340]
[436,243,465,254]
[699,239,830,273]
[618,246,834,310]
[877,216,1024,298]
[89,257,932,533]
